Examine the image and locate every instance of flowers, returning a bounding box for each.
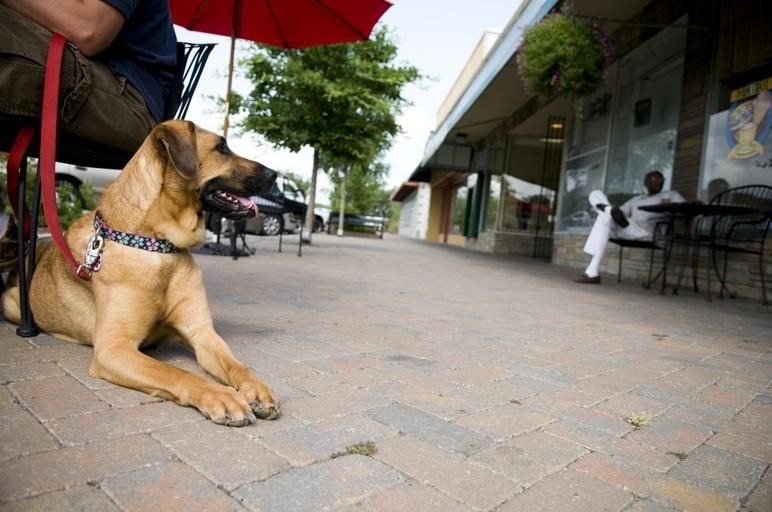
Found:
[514,1,618,123]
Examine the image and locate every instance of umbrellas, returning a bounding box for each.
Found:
[164,1,399,142]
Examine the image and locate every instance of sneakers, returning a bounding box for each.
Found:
[574,272,601,283]
[611,207,629,228]
[0,215,31,273]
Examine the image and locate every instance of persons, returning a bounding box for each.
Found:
[0,2,181,298]
[572,168,689,283]
[693,176,766,236]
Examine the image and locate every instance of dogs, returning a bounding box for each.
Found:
[0,120,280,426]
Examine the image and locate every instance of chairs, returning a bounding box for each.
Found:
[228,183,306,261]
[1,41,218,336]
[608,184,771,305]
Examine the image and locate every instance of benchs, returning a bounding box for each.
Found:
[326,212,384,239]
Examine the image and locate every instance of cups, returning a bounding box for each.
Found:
[660,191,673,204]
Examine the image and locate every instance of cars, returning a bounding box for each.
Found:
[41,162,125,215]
[249,176,321,236]
